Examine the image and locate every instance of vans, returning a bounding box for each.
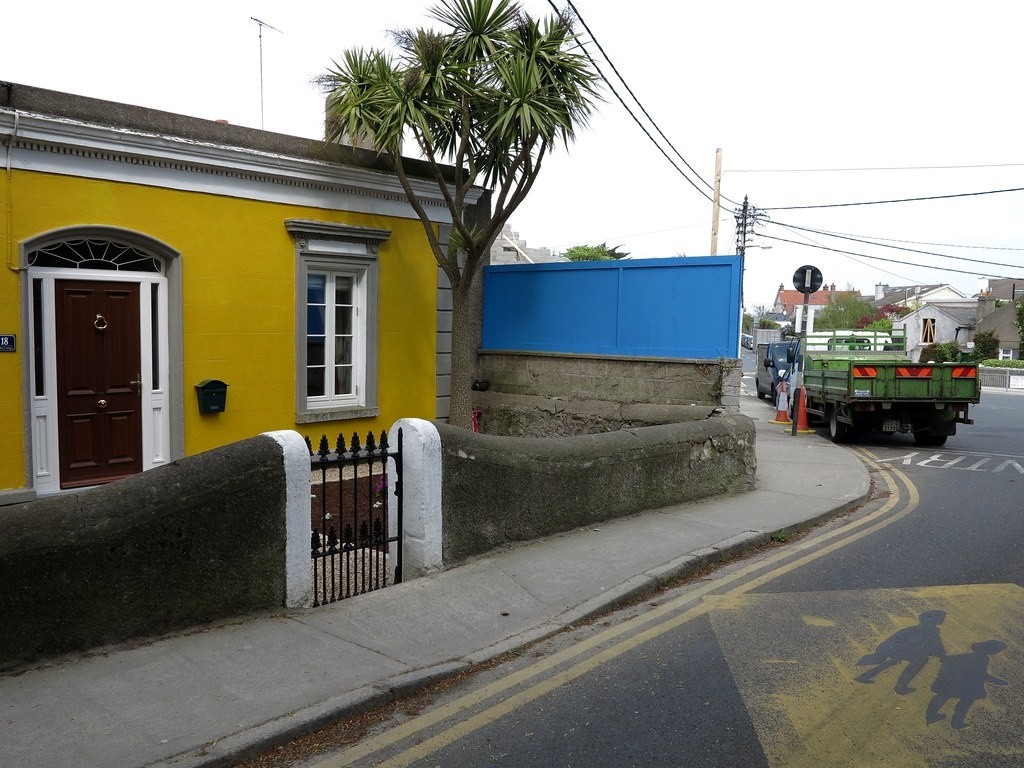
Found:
[754,342,798,405]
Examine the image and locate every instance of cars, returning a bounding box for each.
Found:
[742,333,753,350]
[774,364,793,418]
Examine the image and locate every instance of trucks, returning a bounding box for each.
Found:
[753,329,781,353]
[789,324,983,447]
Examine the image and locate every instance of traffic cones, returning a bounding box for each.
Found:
[768,381,794,425]
[784,386,816,433]
